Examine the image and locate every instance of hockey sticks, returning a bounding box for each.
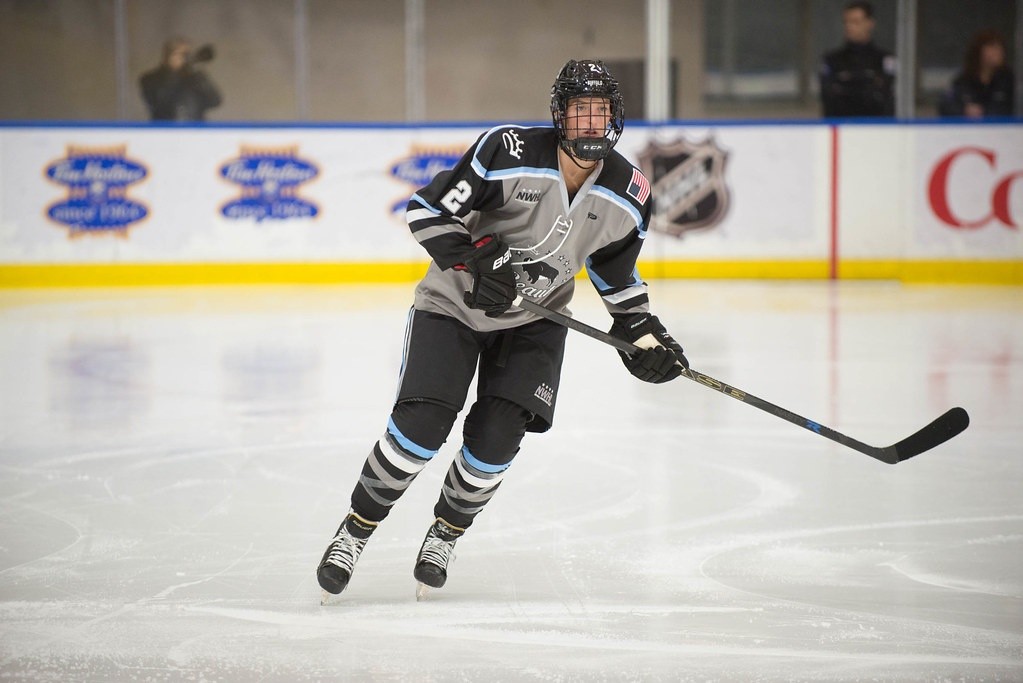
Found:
[512,293,970,464]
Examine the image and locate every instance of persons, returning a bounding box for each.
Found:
[819,0,897,117]
[317,60,689,603]
[939,27,1014,117]
[139,35,221,120]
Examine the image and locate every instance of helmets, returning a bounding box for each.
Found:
[548,58,624,162]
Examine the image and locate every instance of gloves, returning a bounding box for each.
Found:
[608,312,689,384]
[451,233,517,317]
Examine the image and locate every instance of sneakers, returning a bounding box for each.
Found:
[316,505,378,606]
[413,517,465,602]
